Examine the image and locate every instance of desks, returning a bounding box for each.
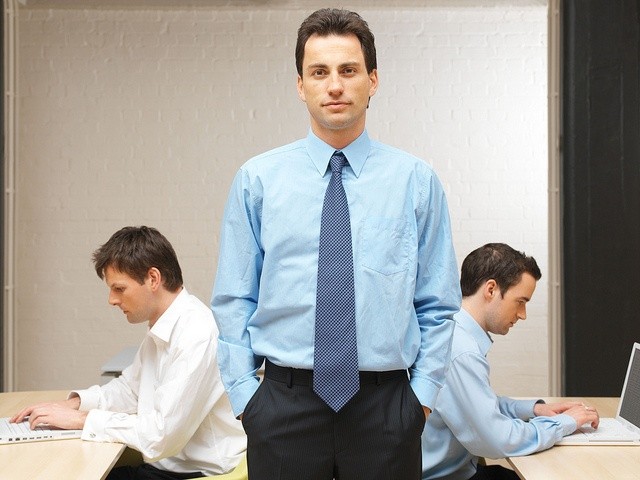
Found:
[100,343,139,380]
[0,389,128,480]
[499,395,640,480]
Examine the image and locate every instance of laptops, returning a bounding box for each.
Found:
[0,413,84,446]
[551,343,640,447]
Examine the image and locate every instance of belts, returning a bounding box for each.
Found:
[264,359,407,386]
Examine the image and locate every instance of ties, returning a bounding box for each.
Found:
[312,155,360,412]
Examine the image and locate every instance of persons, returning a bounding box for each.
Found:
[212,7,464,480]
[9,224,251,479]
[423,240,599,480]
